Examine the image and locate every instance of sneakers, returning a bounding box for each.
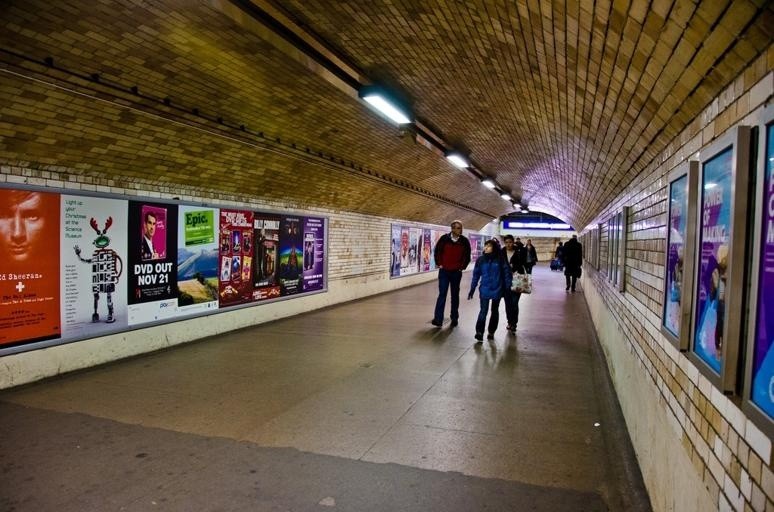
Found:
[450,319,458,327]
[487,333,494,340]
[474,332,484,341]
[430,318,443,326]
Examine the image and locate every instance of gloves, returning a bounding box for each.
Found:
[468,289,474,300]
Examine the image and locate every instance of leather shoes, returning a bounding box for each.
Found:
[507,324,511,329]
[510,326,516,332]
[566,285,570,290]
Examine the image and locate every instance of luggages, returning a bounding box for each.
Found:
[550,256,558,270]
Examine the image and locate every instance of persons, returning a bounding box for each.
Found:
[466,240,513,343]
[140,211,160,260]
[561,235,582,292]
[0,187,51,264]
[430,220,471,327]
[514,237,524,248]
[492,237,501,247]
[390,231,431,277]
[522,238,538,274]
[698,242,719,323]
[709,245,729,350]
[501,234,526,332]
[554,242,564,272]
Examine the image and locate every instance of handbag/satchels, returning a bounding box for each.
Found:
[510,265,533,294]
[578,268,582,278]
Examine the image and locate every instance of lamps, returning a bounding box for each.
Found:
[356,85,416,130]
[445,152,470,168]
